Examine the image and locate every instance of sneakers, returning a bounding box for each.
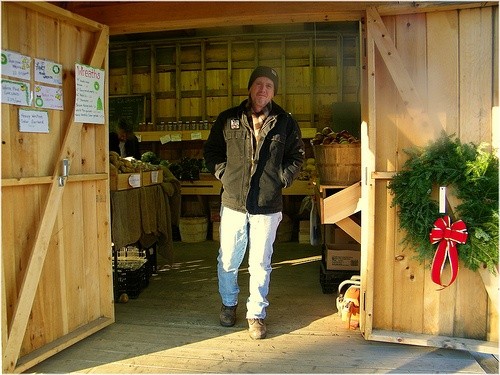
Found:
[220,303,238,326]
[248,319,266,339]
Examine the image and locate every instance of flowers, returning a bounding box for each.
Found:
[299,158,321,215]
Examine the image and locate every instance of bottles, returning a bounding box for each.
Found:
[138,120,217,132]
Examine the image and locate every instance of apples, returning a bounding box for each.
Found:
[312,127,358,145]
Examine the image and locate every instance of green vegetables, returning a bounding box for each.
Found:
[178,156,205,180]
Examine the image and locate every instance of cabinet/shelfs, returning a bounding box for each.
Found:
[180,173,316,195]
[109,22,360,142]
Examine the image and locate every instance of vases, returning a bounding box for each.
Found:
[310,199,320,245]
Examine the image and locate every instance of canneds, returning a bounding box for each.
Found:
[159,120,216,131]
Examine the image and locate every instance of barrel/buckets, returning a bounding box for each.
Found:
[313,143,361,186]
[177,216,208,243]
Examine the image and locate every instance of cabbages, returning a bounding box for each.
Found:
[141,151,178,172]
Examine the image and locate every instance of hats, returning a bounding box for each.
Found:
[248,66,278,96]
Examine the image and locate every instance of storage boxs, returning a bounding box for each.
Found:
[186,195,220,222]
[110,170,163,191]
[112,240,156,299]
[319,224,360,295]
[298,220,310,244]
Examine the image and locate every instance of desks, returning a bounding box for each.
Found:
[110,183,172,247]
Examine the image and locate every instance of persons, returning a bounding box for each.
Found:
[204,66,306,339]
[109,119,141,160]
